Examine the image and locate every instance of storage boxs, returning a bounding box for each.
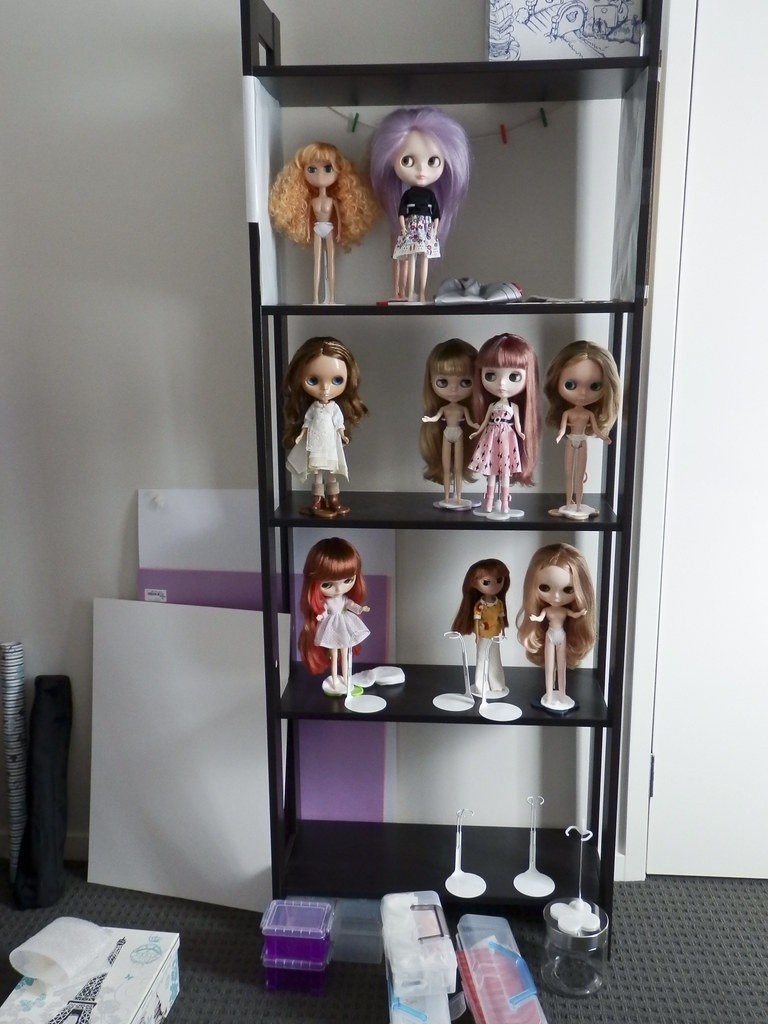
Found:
[331,899,384,964]
[260,940,334,997]
[379,889,459,997]
[456,914,548,1024]
[384,960,452,1023]
[0,927,180,1024]
[260,899,334,961]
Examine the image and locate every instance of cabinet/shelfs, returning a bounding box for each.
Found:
[232,0,665,962]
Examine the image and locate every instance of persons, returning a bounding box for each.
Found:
[267,111,626,717]
